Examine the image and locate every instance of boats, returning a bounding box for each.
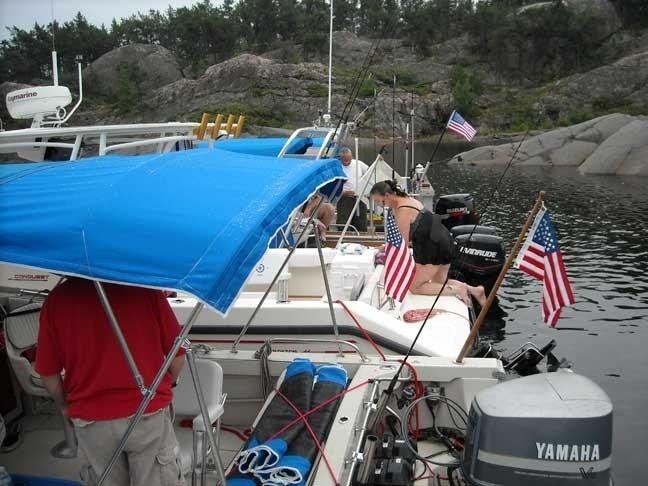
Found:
[1,0,615,486]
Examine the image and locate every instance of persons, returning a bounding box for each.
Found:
[370,180,487,307]
[35,276,186,486]
[336,147,369,232]
[302,195,334,248]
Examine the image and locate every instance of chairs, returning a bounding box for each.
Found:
[3,302,79,458]
[169,358,228,486]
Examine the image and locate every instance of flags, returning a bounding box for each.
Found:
[513,202,575,328]
[447,110,477,142]
[384,208,417,303]
